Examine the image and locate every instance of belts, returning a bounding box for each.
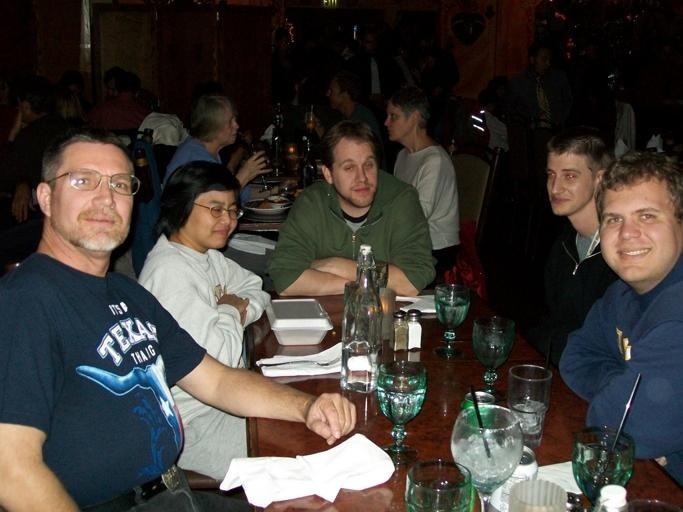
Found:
[78,465,181,512]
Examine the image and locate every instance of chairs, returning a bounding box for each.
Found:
[127,120,191,281]
[440,134,504,274]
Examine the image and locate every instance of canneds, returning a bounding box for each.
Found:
[267,181,280,195]
[460,391,496,441]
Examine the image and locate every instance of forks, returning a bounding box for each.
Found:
[260,356,341,370]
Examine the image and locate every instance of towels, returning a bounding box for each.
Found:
[533,459,583,510]
[393,292,438,315]
[253,339,378,379]
[225,232,278,255]
[213,429,397,511]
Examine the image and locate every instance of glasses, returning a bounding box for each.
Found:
[47,167,140,196]
[194,201,245,221]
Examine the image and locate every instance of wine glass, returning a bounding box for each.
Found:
[430,281,471,357]
[447,403,526,511]
[467,313,516,400]
[373,361,427,469]
[571,428,636,511]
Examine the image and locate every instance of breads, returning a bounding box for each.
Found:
[257,202,272,209]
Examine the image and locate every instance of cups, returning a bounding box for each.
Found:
[507,476,567,512]
[405,462,474,511]
[504,361,552,448]
[339,242,424,396]
[625,498,682,512]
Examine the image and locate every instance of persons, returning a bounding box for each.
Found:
[133,161,273,479]
[0,124,356,512]
[2,42,574,299]
[527,124,623,371]
[558,147,681,493]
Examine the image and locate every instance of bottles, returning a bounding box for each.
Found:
[591,483,632,511]
[244,100,318,217]
